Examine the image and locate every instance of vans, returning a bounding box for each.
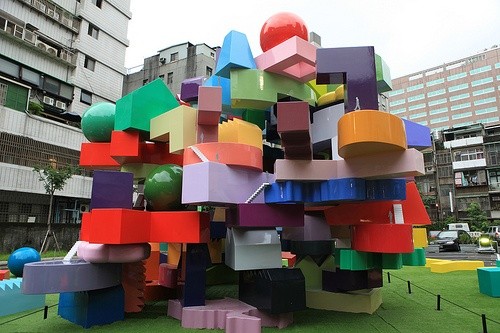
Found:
[483,225,500,244]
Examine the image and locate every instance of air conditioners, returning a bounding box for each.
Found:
[47,7,58,21]
[22,0,32,7]
[159,58,166,62]
[426,165,438,173]
[34,0,45,13]
[44,95,55,104]
[35,41,47,56]
[60,51,72,63]
[78,203,91,213]
[24,29,37,44]
[56,100,66,109]
[60,15,73,28]
[47,44,57,58]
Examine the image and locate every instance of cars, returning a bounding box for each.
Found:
[428,231,441,239]
[429,229,472,252]
